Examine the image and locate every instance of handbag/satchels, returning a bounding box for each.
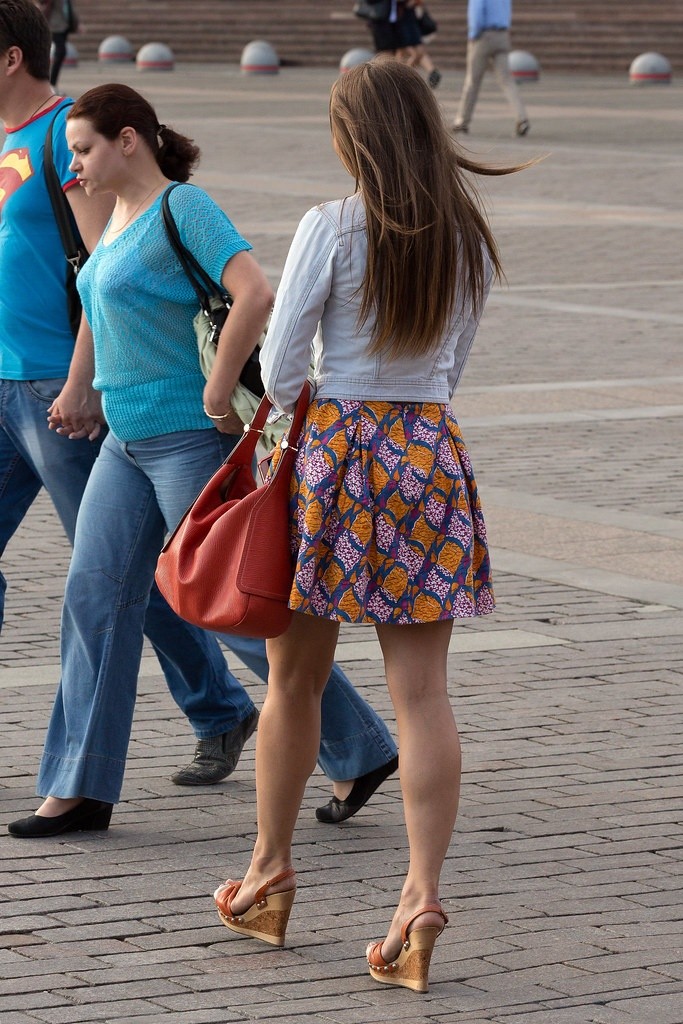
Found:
[317,316,320,317]
[352,0,393,20]
[44,101,91,340]
[415,3,437,36]
[154,380,310,637]
[162,183,319,454]
[66,6,79,32]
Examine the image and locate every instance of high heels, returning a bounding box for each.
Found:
[7,798,114,837]
[315,754,399,824]
[215,868,297,946]
[366,904,448,994]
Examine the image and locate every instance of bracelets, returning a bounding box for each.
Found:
[203,405,232,419]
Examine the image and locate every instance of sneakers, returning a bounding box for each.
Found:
[170,705,259,785]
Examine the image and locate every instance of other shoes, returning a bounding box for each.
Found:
[428,69,441,88]
[52,90,65,97]
[451,126,468,134]
[516,122,530,136]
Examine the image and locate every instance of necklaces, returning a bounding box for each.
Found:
[109,180,161,233]
[30,94,57,117]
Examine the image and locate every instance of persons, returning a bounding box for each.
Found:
[0,0,259,785]
[213,55,508,994]
[447,0,530,136]
[9,84,400,839]
[35,0,79,94]
[353,0,442,88]
